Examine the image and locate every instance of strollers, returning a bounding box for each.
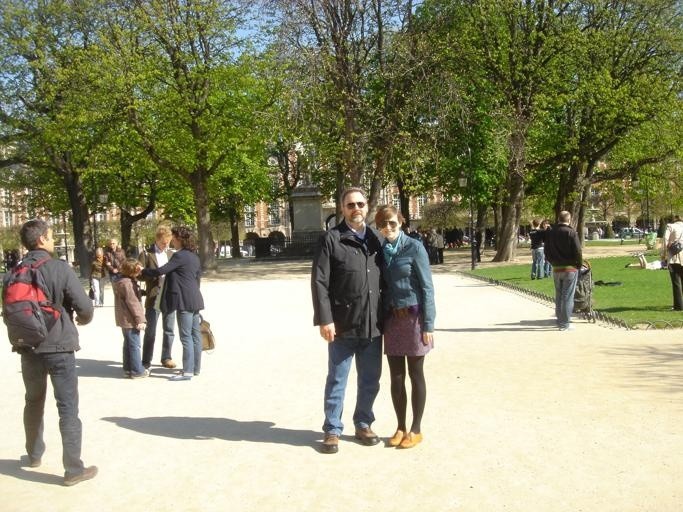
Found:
[571,260,596,323]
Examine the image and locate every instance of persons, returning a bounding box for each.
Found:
[104,238,127,282]
[112,257,148,380]
[374,203,437,449]
[311,185,385,454]
[1,219,99,487]
[409,210,683,331]
[137,226,205,382]
[136,224,178,369]
[89,247,111,308]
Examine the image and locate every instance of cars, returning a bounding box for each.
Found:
[618,227,647,239]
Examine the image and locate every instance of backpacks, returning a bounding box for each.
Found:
[1,253,60,350]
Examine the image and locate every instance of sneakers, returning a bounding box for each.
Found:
[160,357,175,369]
[400,432,423,448]
[122,360,151,379]
[388,430,407,446]
[167,373,191,381]
[321,432,339,453]
[354,426,380,446]
[177,368,198,377]
[63,465,98,486]
[27,456,41,467]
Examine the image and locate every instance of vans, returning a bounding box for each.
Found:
[586,221,609,240]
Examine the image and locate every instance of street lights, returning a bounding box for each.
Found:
[89,174,109,249]
[632,168,650,227]
[455,146,474,272]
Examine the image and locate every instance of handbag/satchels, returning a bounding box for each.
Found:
[671,241,682,254]
[88,281,94,300]
[194,315,215,351]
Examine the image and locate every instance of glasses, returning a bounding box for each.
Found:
[342,202,367,209]
[378,220,397,229]
